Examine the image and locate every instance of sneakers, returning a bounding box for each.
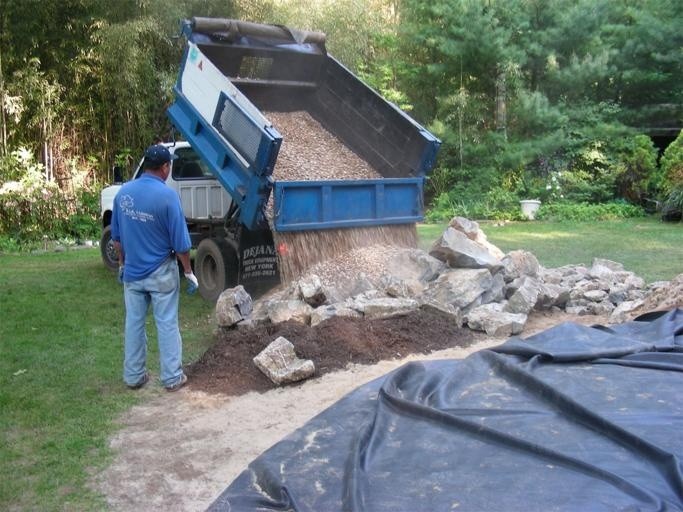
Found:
[166,373,188,392]
[127,372,151,389]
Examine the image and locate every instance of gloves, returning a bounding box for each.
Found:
[117,265,124,284]
[183,269,199,295]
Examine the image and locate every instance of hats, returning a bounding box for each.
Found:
[143,144,179,164]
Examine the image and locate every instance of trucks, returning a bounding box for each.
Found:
[98,17,443,303]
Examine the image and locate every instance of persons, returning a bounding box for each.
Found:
[108,144,200,395]
